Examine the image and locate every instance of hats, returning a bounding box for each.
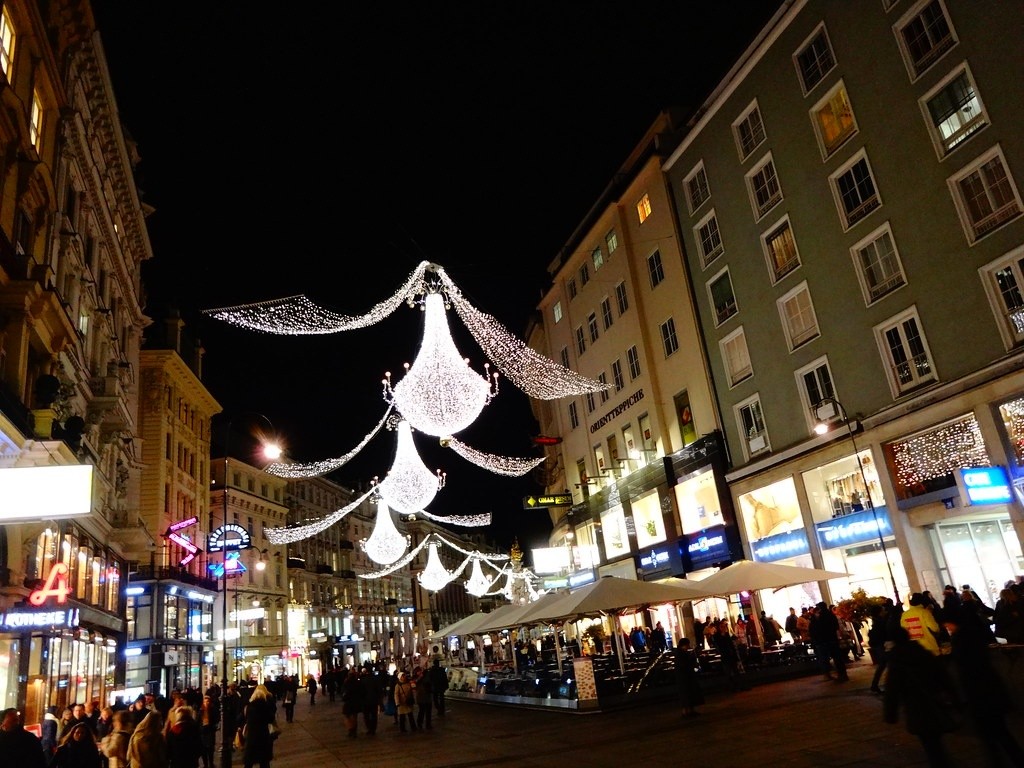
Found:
[398,672,407,681]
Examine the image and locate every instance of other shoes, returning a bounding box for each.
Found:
[418,726,424,729]
[839,670,850,683]
[400,729,409,732]
[345,727,357,738]
[439,710,446,715]
[412,726,418,731]
[685,711,701,721]
[393,720,399,724]
[366,730,376,736]
[426,725,434,729]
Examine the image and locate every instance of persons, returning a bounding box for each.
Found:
[306,578,1024,737]
[0,673,300,768]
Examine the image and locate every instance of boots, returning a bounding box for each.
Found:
[870,672,881,691]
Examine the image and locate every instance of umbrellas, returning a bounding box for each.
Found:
[421,561,855,640]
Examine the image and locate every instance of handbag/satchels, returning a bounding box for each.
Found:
[405,695,415,706]
[269,719,282,737]
[235,730,245,748]
